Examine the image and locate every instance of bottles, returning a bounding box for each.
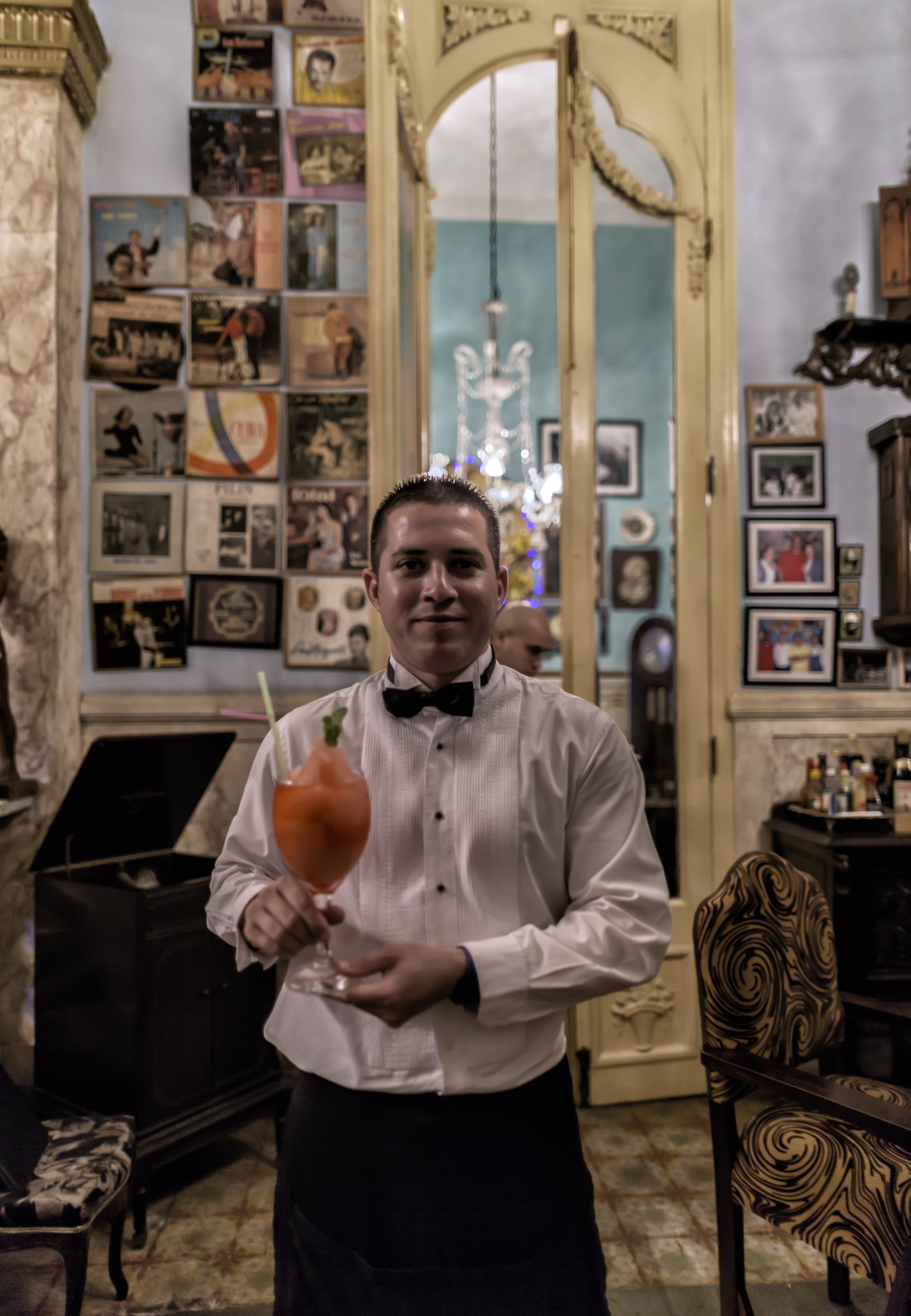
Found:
[804,731,881,816]
[888,730,911,837]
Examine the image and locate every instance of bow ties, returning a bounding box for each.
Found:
[381,644,496,719]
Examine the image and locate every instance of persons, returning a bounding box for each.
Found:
[324,304,354,380]
[105,227,161,286]
[292,417,365,477]
[103,601,184,667]
[490,605,554,677]
[297,142,364,187]
[102,511,168,556]
[188,119,280,197]
[286,206,328,289]
[204,472,671,1316]
[102,406,148,468]
[214,306,265,381]
[287,492,368,571]
[300,49,360,107]
[755,389,832,677]
[196,201,255,287]
[334,624,368,668]
[216,501,278,571]
[91,319,184,383]
[297,583,364,634]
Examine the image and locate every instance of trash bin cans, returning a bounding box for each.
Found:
[27,725,276,1174]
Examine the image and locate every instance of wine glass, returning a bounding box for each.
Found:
[269,722,374,993]
